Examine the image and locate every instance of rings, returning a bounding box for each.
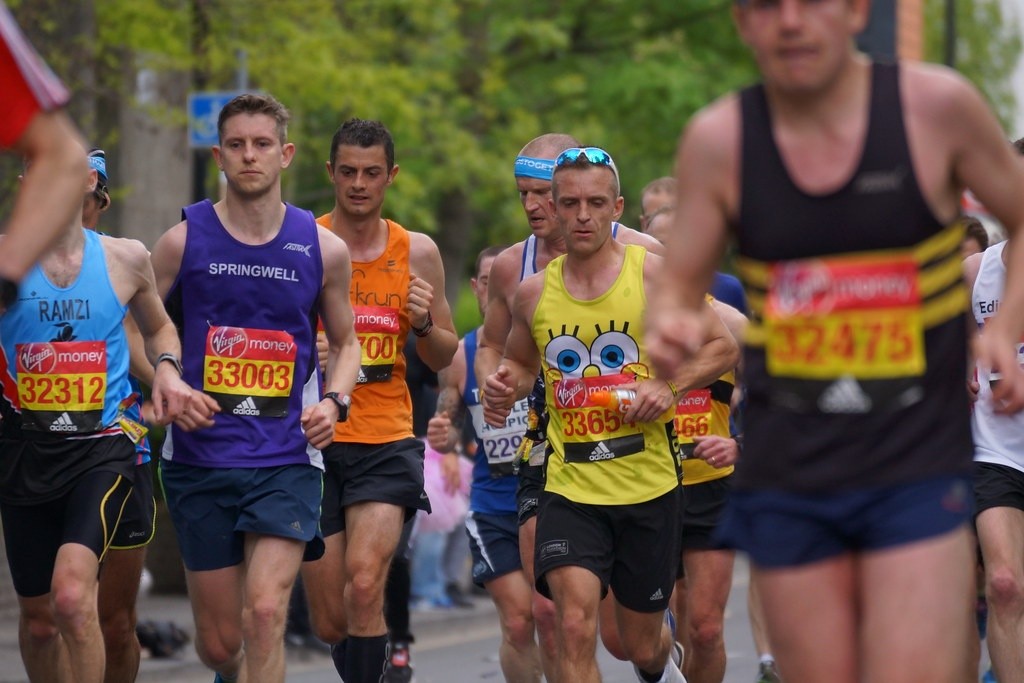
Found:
[711,456,716,463]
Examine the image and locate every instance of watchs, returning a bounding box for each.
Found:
[156,354,183,376]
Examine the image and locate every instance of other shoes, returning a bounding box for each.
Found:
[670,641,685,672]
[753,654,780,683]
[445,581,476,609]
[632,652,688,683]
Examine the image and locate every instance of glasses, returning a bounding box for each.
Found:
[554,146,621,197]
[642,204,678,228]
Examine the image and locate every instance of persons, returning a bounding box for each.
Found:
[86,149,111,237]
[966,139,1024,683]
[0,148,194,683]
[960,215,988,260]
[303,118,459,683]
[0,0,90,318]
[142,92,362,683]
[645,0,1024,683]
[474,132,746,682]
[426,247,546,682]
[377,334,440,683]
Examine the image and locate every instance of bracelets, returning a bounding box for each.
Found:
[323,393,351,423]
[411,313,433,336]
[0,275,17,310]
[479,389,485,402]
[665,377,678,398]
